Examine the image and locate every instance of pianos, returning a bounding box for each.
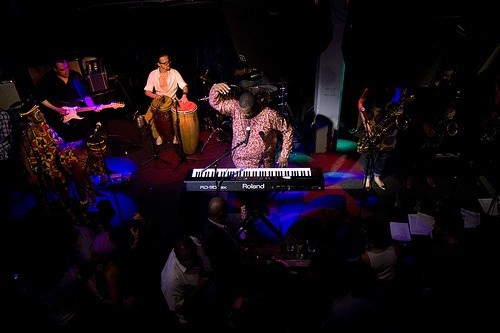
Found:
[183,166,325,192]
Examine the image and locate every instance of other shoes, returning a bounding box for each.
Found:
[363,176,371,192]
[373,176,386,191]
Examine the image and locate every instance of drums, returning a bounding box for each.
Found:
[151,95,173,148]
[246,86,265,107]
[262,84,278,102]
[172,100,201,157]
[223,85,243,100]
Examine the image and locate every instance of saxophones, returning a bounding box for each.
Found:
[357,88,416,153]
[422,88,464,152]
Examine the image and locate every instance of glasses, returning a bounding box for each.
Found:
[160,61,171,65]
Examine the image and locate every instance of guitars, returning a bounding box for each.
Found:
[58,100,125,123]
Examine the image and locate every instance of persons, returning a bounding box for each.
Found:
[24,191,451,330]
[5,73,68,177]
[45,58,95,144]
[405,60,469,189]
[358,74,406,192]
[208,81,294,178]
[141,52,188,134]
[1,107,13,169]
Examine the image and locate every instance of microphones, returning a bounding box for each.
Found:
[244,126,251,146]
[174,97,180,106]
[359,107,370,123]
[90,120,101,138]
[160,96,165,104]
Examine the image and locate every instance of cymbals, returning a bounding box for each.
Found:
[271,78,290,84]
